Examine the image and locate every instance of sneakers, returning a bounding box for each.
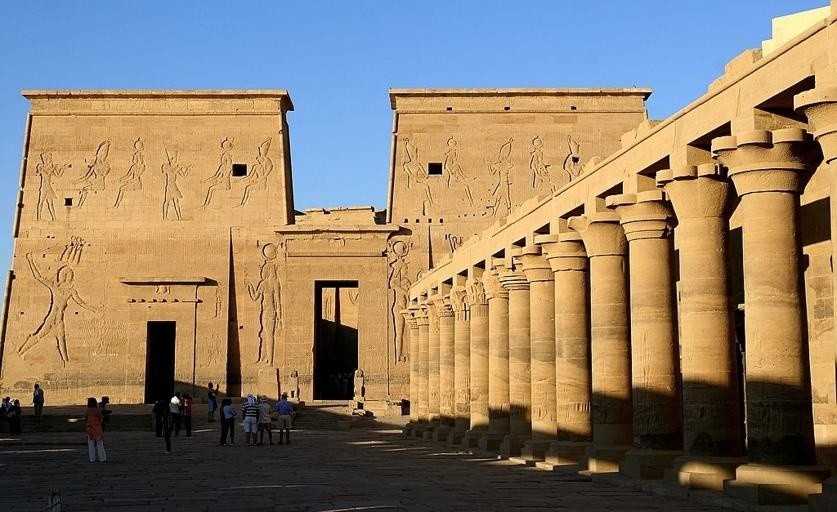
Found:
[219,441,292,447]
[160,451,171,456]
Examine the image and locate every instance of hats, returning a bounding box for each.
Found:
[246,392,290,402]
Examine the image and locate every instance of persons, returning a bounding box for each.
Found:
[5,400,22,438]
[150,382,298,455]
[30,384,44,431]
[83,398,111,465]
[2,398,8,413]
[1,397,11,420]
[95,396,112,445]
